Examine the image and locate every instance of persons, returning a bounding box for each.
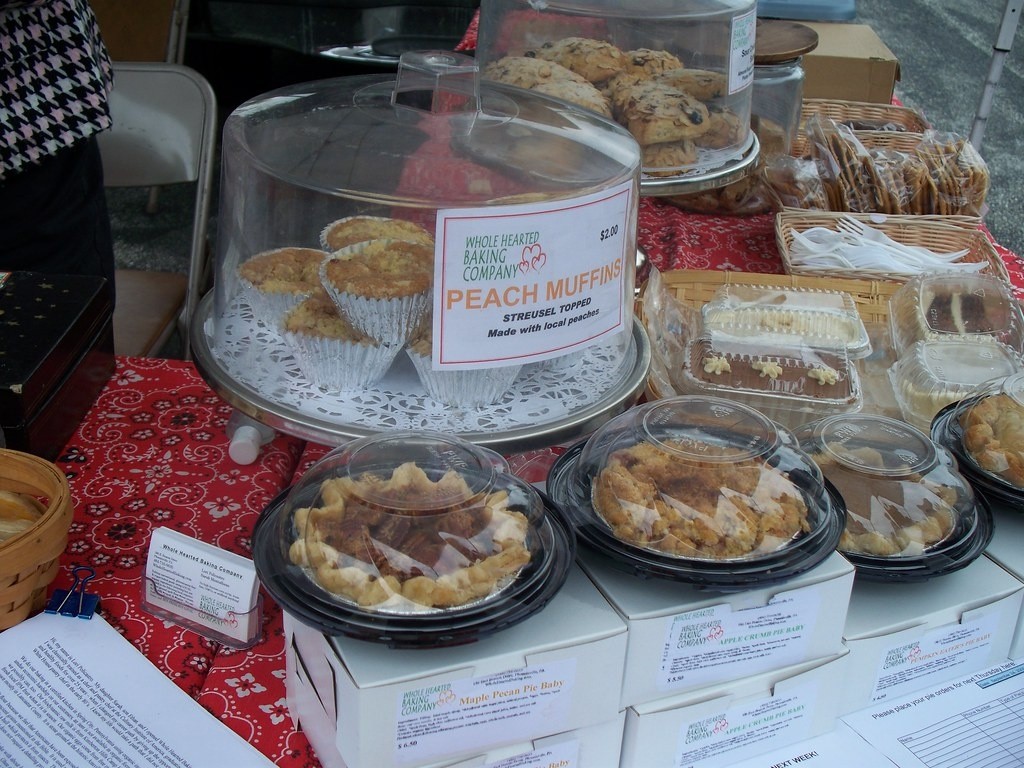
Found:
[0,0,114,273]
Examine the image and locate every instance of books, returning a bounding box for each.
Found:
[0,609,281,768]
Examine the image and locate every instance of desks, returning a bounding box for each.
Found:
[194,0,1024,768]
[41,357,303,693]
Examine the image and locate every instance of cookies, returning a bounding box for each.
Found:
[693,115,990,215]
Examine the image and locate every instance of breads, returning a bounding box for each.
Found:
[481,36,747,181]
[238,217,1024,608]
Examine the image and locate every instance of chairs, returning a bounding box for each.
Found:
[95,0,222,359]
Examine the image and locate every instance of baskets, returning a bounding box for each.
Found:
[0,447,73,632]
[635,95,1012,444]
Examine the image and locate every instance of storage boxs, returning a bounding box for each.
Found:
[286,479,1024,768]
[0,267,118,461]
[793,21,900,106]
